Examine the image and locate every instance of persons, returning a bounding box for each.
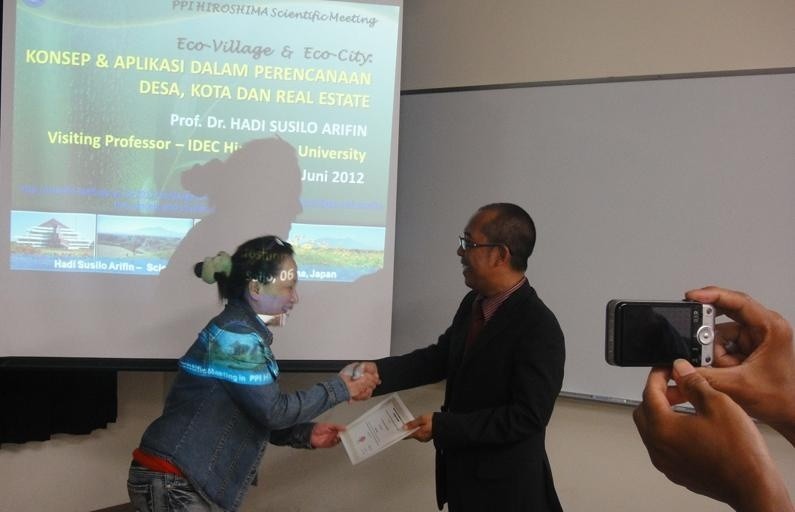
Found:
[332,199,568,510]
[124,232,382,510]
[629,284,795,511]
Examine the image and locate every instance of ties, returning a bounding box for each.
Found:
[466,301,483,344]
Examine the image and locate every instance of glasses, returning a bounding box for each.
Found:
[244,237,284,272]
[458,235,500,250]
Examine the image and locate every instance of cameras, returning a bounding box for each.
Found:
[604,298,716,367]
